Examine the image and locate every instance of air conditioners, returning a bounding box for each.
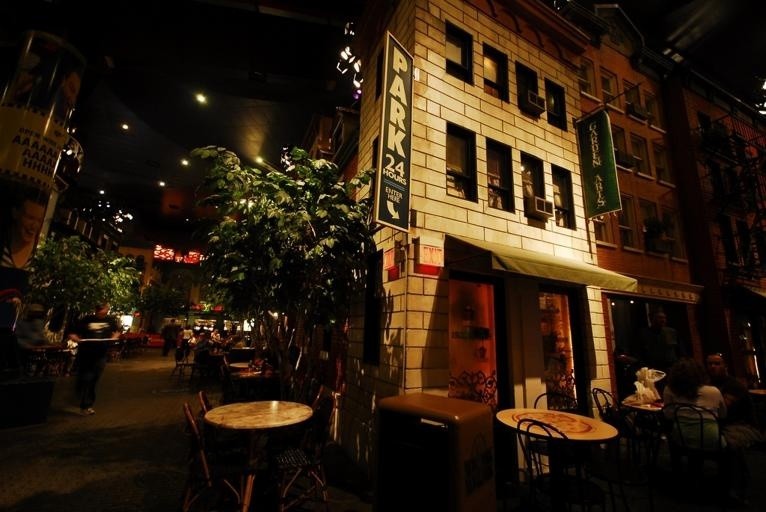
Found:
[525,195,554,219]
[520,91,546,116]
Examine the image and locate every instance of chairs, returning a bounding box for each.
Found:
[168,348,338,512]
[513,386,748,511]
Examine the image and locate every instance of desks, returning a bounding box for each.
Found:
[495,408,620,512]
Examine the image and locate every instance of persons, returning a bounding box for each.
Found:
[32,300,125,415]
[541,318,567,380]
[161,318,239,383]
[637,309,763,495]
[0,186,48,273]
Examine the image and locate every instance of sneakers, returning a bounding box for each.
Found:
[64,407,95,416]
